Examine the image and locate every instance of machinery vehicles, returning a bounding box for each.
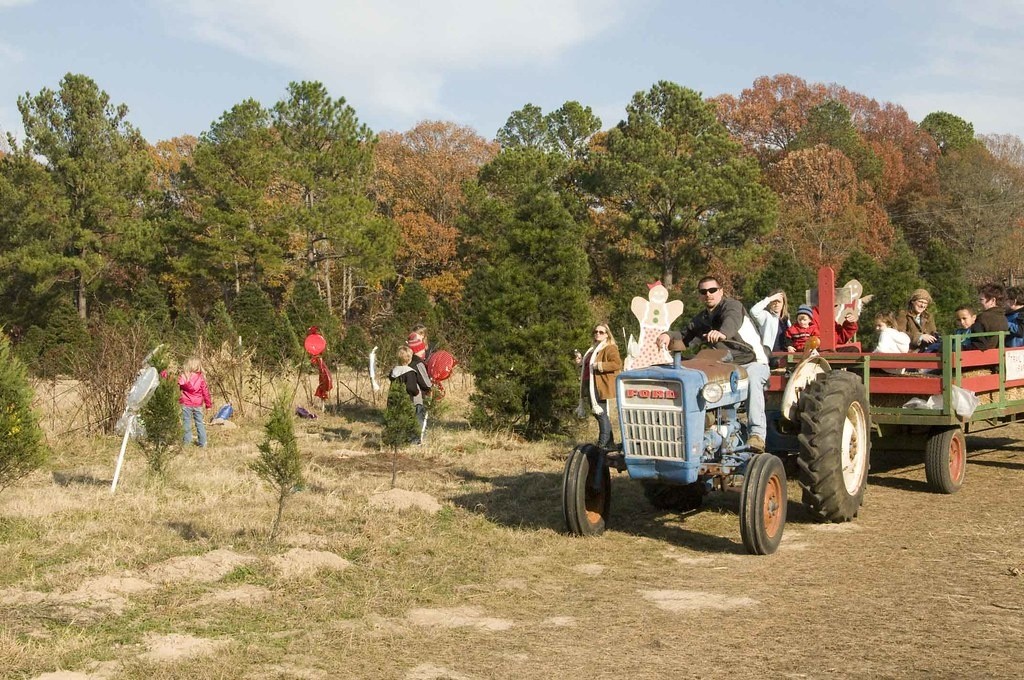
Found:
[562,266,1024,555]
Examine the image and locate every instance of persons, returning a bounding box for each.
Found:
[388,346,420,407]
[656,277,772,453]
[573,323,622,450]
[412,324,446,399]
[866,310,912,376]
[812,300,857,349]
[783,304,821,353]
[1005,286,1024,348]
[750,289,793,353]
[896,288,939,373]
[160,356,213,447]
[406,332,431,447]
[959,283,1009,372]
[936,303,979,374]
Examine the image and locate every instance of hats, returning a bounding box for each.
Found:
[405,331,425,354]
[908,289,931,304]
[796,304,812,318]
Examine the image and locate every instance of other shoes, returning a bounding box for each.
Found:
[746,435,765,454]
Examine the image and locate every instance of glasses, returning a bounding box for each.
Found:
[593,330,607,335]
[699,287,721,295]
